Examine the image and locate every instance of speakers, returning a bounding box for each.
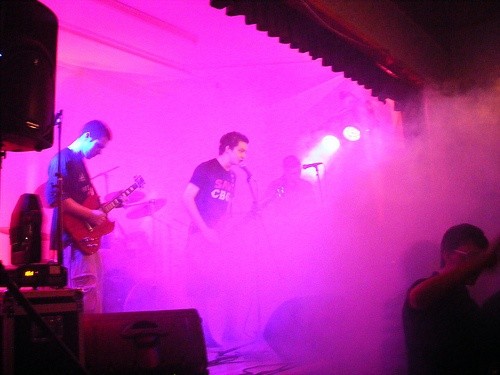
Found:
[1,0,61,152]
[83,307,210,374]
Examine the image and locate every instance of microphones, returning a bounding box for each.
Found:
[302,162,323,169]
[240,164,249,176]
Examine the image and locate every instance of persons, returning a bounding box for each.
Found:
[256,155,317,217]
[182,131,250,246]
[48,120,122,313]
[377,239,441,374]
[398,221,499,375]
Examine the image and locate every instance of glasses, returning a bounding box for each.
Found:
[451,248,477,259]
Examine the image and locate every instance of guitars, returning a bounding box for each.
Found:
[58,174,145,256]
[189,183,288,273]
